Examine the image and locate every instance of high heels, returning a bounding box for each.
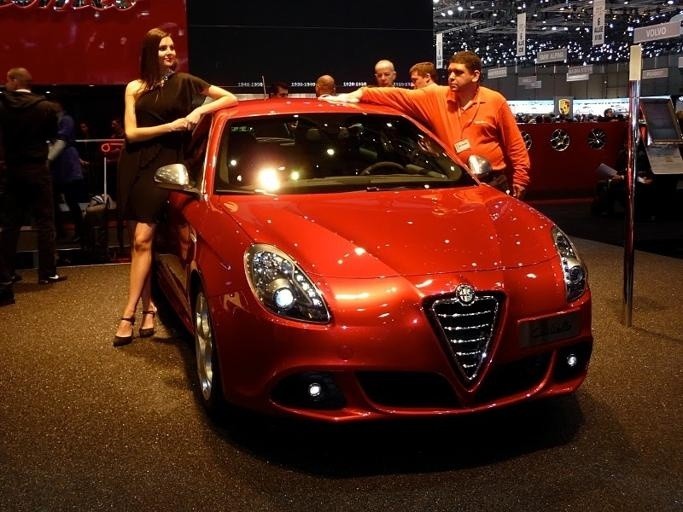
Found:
[138,306,157,336]
[114,315,135,346]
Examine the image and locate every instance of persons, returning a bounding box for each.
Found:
[114,28,238,346]
[515,109,653,188]
[331,51,531,201]
[268,81,290,101]
[315,74,338,98]
[374,59,398,87]
[0,67,123,285]
[407,62,438,89]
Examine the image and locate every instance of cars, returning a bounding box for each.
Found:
[588,146,682,257]
[149,98,593,431]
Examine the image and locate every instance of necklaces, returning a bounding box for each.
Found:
[155,70,175,81]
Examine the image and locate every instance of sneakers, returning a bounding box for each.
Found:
[0,274,22,285]
[39,273,67,283]
